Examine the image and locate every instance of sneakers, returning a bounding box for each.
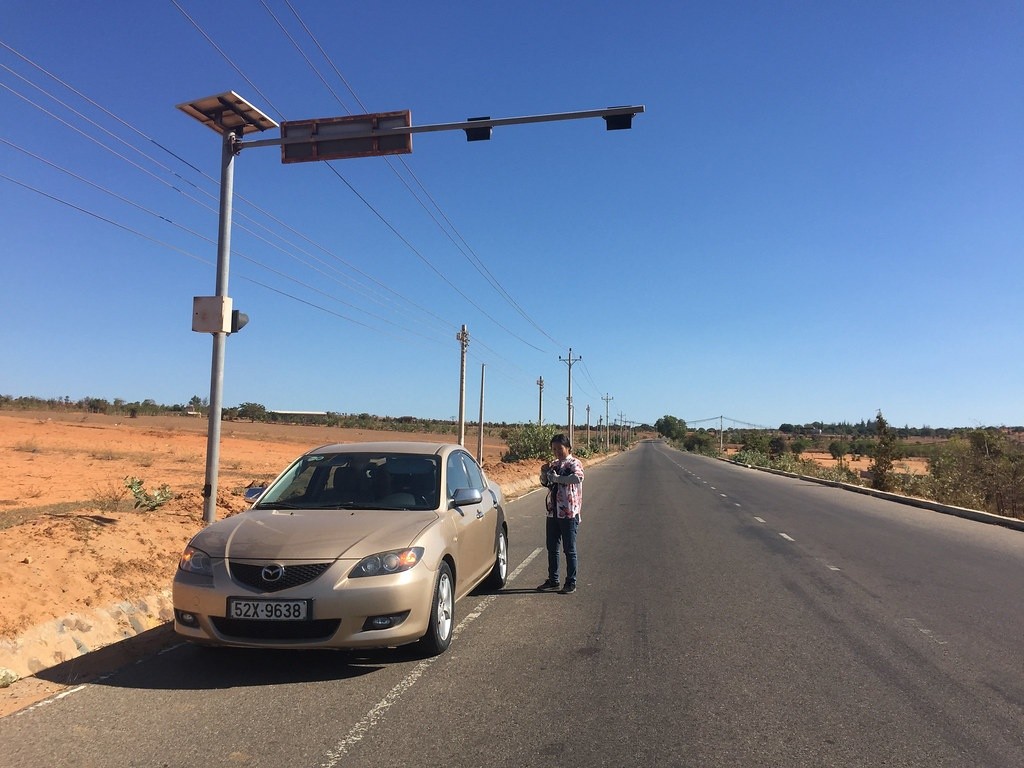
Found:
[538,579,560,590]
[560,578,576,593]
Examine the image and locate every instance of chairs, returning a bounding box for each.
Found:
[318,467,366,502]
[383,459,436,506]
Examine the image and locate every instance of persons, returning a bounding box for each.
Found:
[537,433,584,594]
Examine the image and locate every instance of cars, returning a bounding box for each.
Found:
[170,438,513,660]
